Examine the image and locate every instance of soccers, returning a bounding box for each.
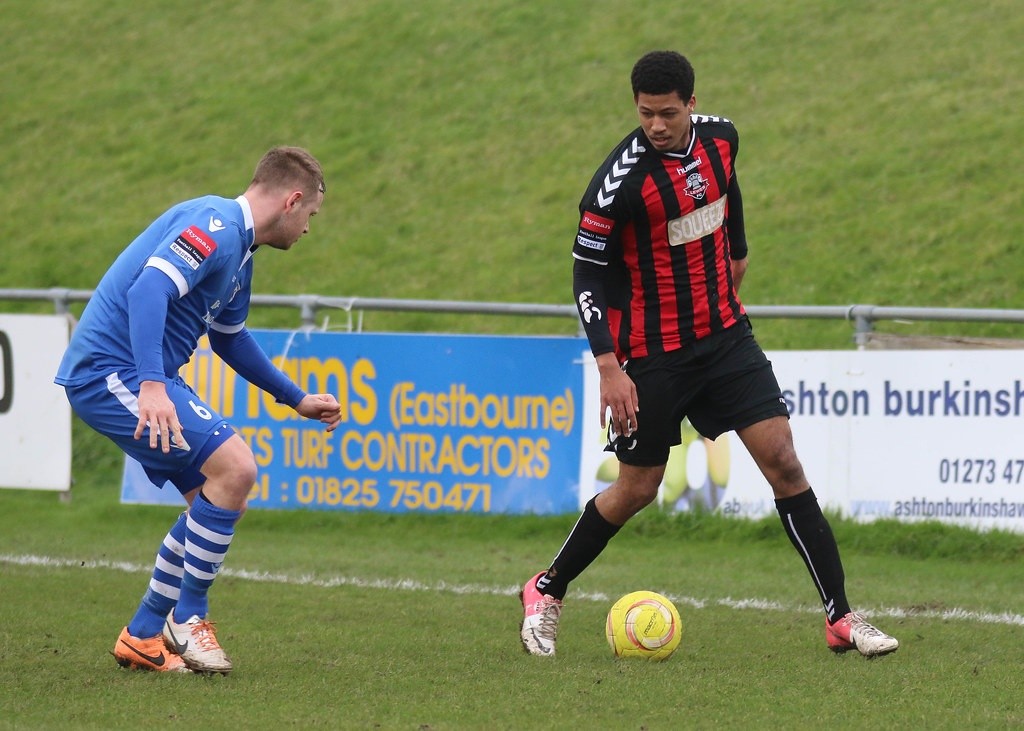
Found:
[604,589,682,662]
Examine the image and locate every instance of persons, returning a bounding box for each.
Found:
[518,50,900,657]
[53,148,342,675]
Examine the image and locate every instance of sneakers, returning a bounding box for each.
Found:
[161,607,233,674]
[113,627,194,674]
[520,571,563,657]
[825,611,899,655]
